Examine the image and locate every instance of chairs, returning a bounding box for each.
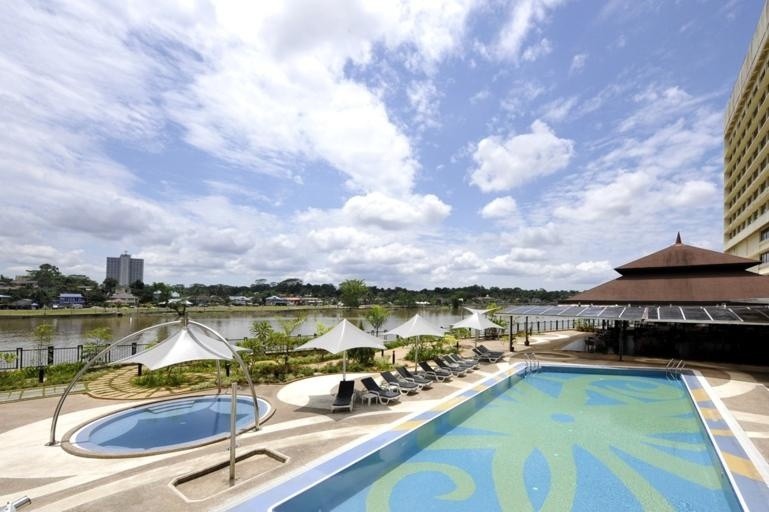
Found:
[330,344,506,415]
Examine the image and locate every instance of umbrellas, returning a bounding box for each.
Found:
[452,312,506,360]
[292,317,388,381]
[381,314,447,375]
